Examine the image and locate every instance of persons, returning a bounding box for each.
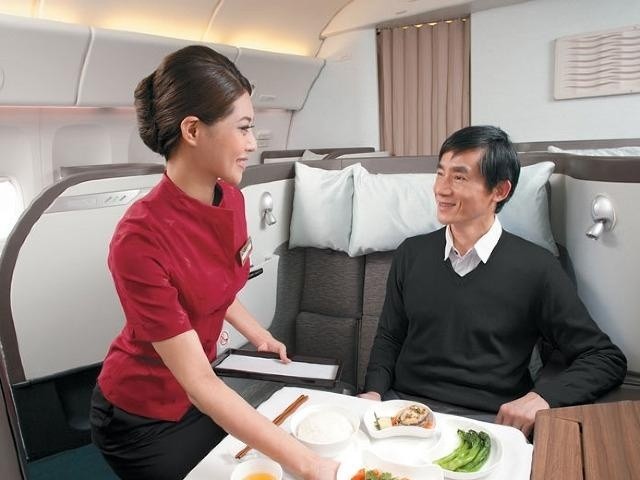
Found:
[86,43,342,480]
[350,123,631,444]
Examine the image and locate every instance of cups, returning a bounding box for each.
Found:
[231,456,282,480]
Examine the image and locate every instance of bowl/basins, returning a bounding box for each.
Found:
[289,399,505,480]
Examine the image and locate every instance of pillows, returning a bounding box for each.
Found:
[349,159,559,256]
[289,162,360,251]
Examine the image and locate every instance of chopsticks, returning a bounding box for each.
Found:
[228,391,310,460]
